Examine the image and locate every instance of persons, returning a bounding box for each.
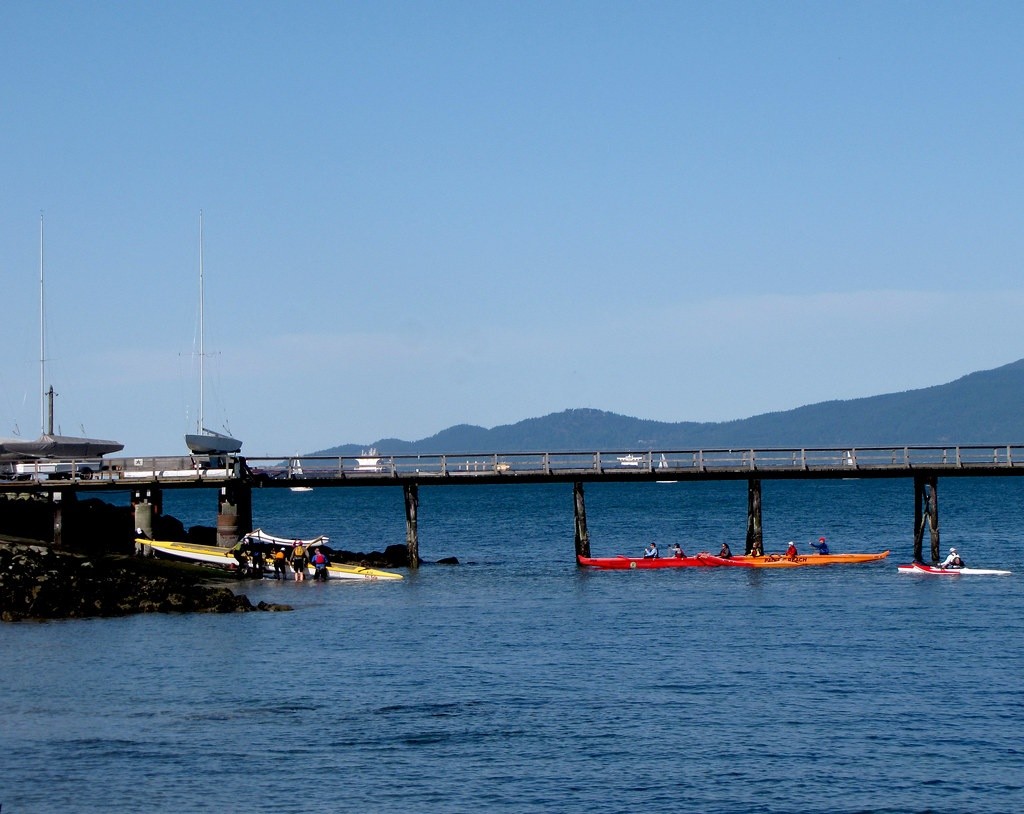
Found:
[133,528,152,555]
[808,537,829,555]
[670,543,684,558]
[747,541,761,557]
[785,541,797,557]
[937,547,966,569]
[233,539,332,582]
[720,542,732,558]
[644,542,659,558]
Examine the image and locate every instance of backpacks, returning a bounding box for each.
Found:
[295,546,303,556]
[316,554,324,563]
[276,552,284,559]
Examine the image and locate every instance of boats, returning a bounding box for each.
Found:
[135,537,405,579]
[895,560,1014,579]
[577,553,707,568]
[699,549,890,569]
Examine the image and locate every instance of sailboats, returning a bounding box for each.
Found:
[184,206,247,454]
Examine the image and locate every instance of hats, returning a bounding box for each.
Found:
[299,542,303,545]
[281,548,285,550]
